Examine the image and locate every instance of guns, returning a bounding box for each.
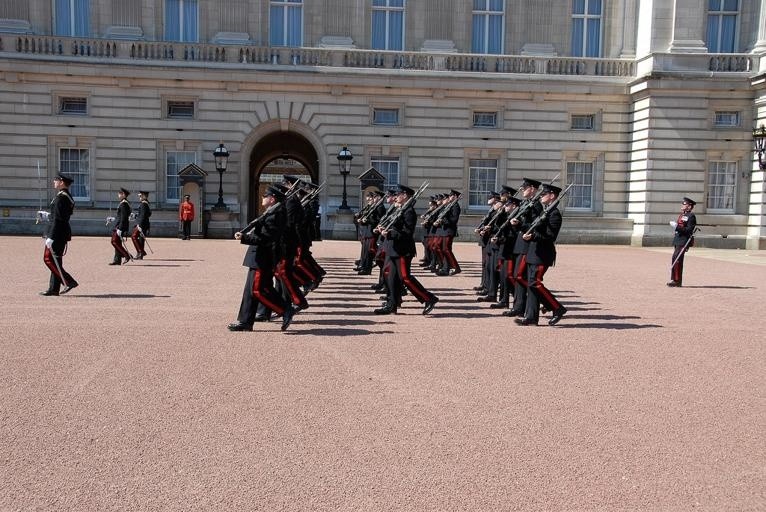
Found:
[239,177,326,235]
[476,171,575,237]
[356,179,464,241]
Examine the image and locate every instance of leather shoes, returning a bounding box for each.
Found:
[474,287,567,326]
[39,289,59,296]
[60,281,78,294]
[372,284,438,315]
[123,255,132,264]
[419,259,460,275]
[353,260,371,275]
[109,261,121,265]
[134,251,146,260]
[667,280,681,287]
[227,271,326,330]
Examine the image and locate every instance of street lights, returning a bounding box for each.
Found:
[209,145,235,207]
[748,123,765,171]
[332,145,358,210]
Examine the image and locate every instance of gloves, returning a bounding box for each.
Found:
[46,238,54,248]
[38,211,50,221]
[117,229,122,236]
[670,221,678,228]
[107,217,115,221]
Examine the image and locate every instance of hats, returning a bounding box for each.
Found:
[367,184,414,196]
[682,197,696,205]
[118,188,130,196]
[137,190,149,196]
[54,172,74,183]
[488,178,562,205]
[263,174,319,199]
[429,189,461,202]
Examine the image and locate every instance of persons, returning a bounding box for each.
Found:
[36,171,80,296]
[419,189,461,276]
[667,196,696,286]
[130,190,152,261]
[107,187,133,265]
[179,194,194,240]
[228,176,327,330]
[353,190,386,275]
[371,184,438,315]
[474,178,568,325]
